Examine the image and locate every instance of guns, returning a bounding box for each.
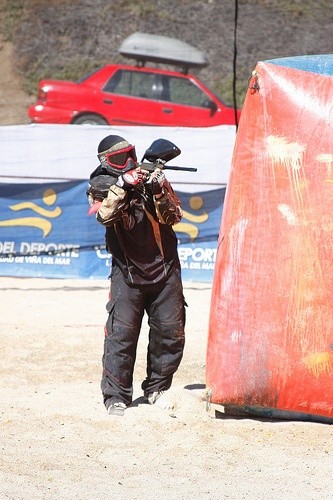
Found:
[133,138,198,188]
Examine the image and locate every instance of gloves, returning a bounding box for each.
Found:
[115,166,150,190]
[145,166,165,194]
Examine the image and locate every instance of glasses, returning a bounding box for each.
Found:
[105,145,137,168]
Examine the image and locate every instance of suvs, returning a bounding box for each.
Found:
[29,32,242,127]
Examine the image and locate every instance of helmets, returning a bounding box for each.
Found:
[98,135,137,175]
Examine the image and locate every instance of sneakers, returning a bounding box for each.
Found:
[144,389,176,410]
[107,402,125,416]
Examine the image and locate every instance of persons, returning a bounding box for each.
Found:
[86,135,197,415]
